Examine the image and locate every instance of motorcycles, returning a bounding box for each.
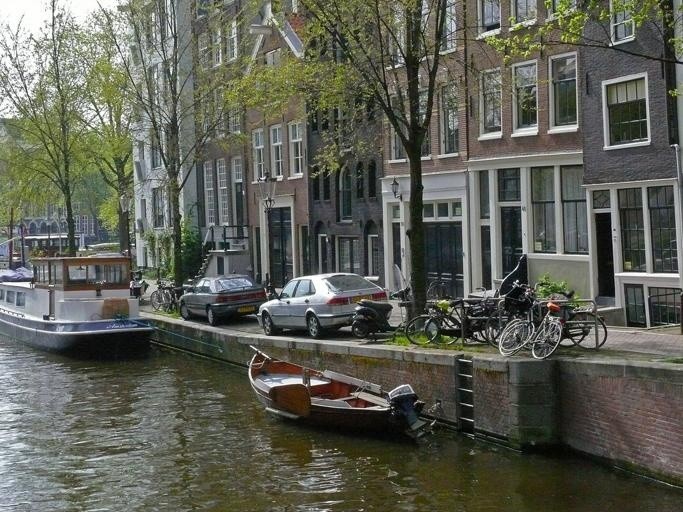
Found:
[348,287,416,336]
[128,267,147,302]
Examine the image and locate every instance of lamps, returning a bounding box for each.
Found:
[389,176,404,203]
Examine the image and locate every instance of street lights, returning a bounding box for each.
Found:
[254,170,280,299]
[116,193,133,286]
[16,222,28,271]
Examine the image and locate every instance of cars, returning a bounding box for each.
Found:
[179,274,268,326]
[259,272,389,335]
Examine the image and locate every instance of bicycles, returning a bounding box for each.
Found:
[150,280,176,315]
[402,285,612,357]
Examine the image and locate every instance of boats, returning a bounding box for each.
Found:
[242,343,433,443]
[0,251,158,355]
